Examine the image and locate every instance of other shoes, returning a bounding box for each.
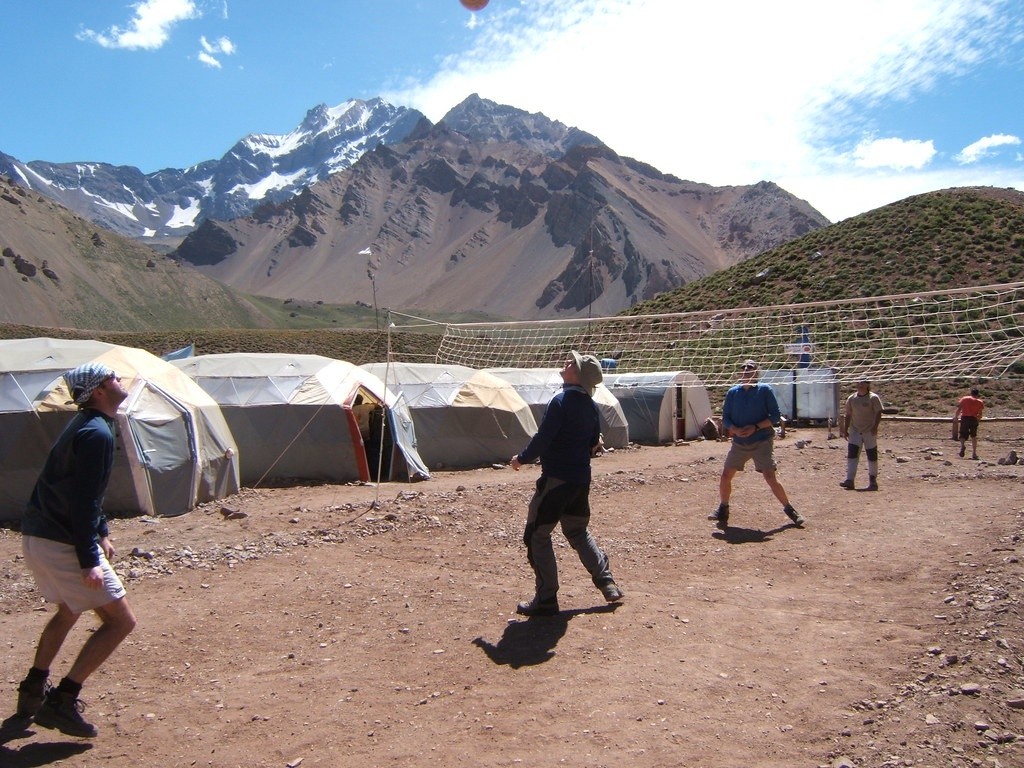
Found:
[865,484,878,491]
[959,447,966,457]
[593,578,625,601]
[973,454,977,459]
[519,590,558,615]
[840,479,855,489]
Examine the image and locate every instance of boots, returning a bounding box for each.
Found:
[707,504,730,520]
[784,504,804,526]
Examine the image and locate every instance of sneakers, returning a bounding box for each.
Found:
[34,688,98,737]
[18,678,56,715]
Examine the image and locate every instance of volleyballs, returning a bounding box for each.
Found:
[458,0,489,11]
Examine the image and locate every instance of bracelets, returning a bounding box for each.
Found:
[755,423,761,432]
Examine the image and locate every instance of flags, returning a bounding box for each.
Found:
[794,328,811,368]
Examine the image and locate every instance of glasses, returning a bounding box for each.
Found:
[101,370,117,384]
[742,365,753,370]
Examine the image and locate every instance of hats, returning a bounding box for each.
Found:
[571,349,603,396]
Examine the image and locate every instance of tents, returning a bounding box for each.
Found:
[359,362,539,471]
[165,353,431,486]
[478,367,629,449]
[601,370,712,444]
[0,340,240,519]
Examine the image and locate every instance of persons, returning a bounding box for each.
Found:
[352,392,378,468]
[17,362,136,739]
[839,375,884,491]
[954,388,984,460]
[509,349,625,617]
[707,359,805,526]
